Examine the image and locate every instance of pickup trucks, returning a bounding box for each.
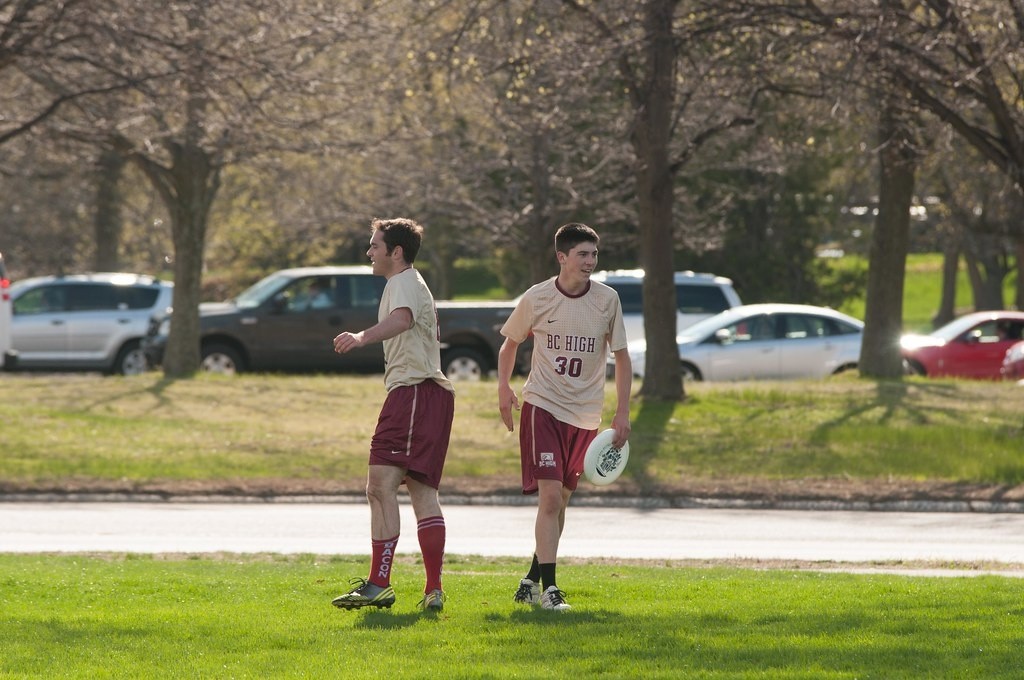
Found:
[140,266,533,381]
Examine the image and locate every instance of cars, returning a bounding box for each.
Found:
[606,303,864,379]
[898,309,1023,378]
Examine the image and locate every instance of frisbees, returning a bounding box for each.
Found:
[583,429,630,486]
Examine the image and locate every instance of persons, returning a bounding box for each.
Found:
[38,289,58,308]
[332,218,456,611]
[290,283,330,309]
[995,325,1008,341]
[498,223,632,610]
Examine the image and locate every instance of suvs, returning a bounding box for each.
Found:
[1,268,173,376]
[509,267,742,352]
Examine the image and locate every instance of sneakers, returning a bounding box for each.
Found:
[332,576,396,610]
[514,578,542,607]
[415,588,446,612]
[541,584,572,611]
